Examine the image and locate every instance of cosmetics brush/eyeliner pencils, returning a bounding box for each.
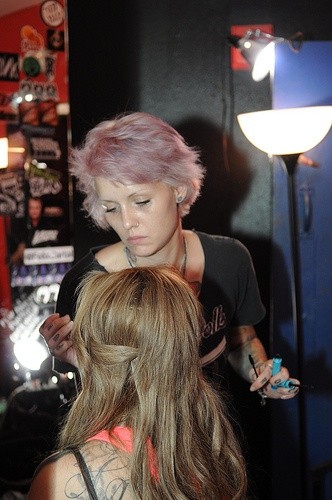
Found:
[248,355,266,406]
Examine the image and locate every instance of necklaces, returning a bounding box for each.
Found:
[123,241,187,281]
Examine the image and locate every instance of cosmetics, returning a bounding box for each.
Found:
[271,353,313,392]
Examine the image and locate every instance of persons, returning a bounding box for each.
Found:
[9,198,71,263]
[22,268,246,500]
[38,111,300,417]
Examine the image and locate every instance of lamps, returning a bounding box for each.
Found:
[235,104,332,500]
[226,35,275,234]
